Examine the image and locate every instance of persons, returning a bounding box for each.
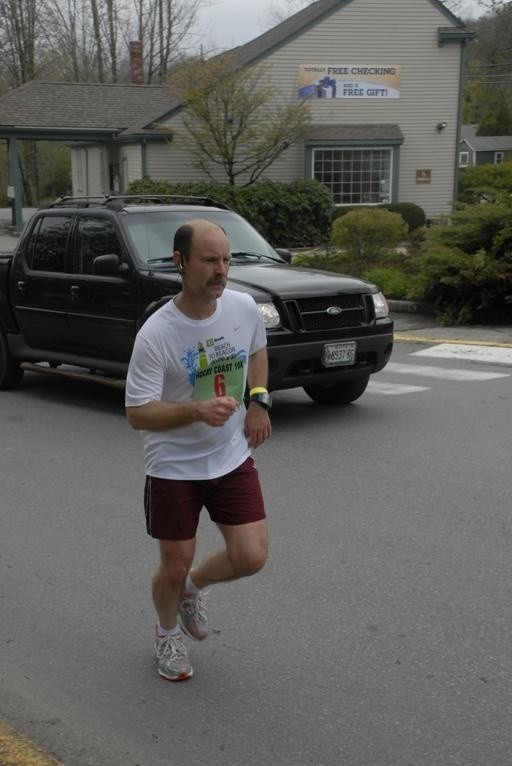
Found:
[124,220,276,682]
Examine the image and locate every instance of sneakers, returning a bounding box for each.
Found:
[153,623,194,680]
[177,587,213,640]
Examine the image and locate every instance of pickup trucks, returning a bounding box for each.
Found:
[0,194,395,410]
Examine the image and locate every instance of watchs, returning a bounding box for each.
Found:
[249,392,273,411]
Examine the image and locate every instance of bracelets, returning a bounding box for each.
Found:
[249,387,267,397]
